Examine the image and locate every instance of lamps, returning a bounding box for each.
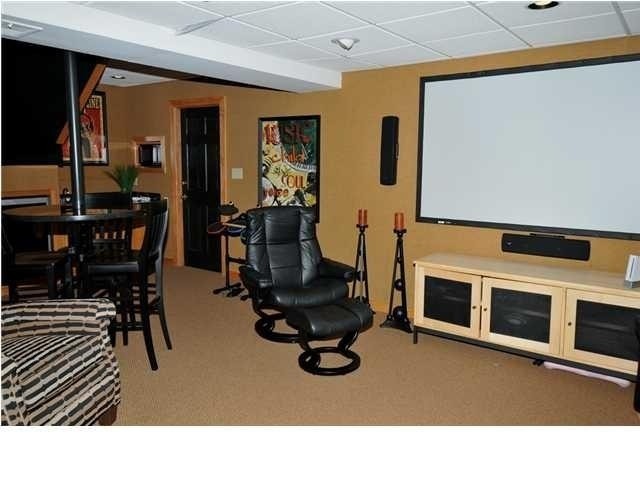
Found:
[336,39,354,51]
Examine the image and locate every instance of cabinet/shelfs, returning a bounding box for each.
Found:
[413,253,640,384]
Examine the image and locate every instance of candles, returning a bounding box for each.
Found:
[359,209,367,225]
[395,212,405,231]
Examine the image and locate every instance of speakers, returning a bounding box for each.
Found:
[501,233,591,261]
[380,116,399,186]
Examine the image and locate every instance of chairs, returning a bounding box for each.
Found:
[1,298,120,426]
[1,191,172,371]
[239,205,355,343]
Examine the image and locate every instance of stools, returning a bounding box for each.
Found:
[285,299,373,376]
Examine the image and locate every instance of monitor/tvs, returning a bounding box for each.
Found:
[415,53,640,242]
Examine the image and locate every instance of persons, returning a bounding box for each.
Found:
[63,111,102,159]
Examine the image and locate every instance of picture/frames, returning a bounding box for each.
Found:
[258,115,321,224]
[58,91,110,167]
